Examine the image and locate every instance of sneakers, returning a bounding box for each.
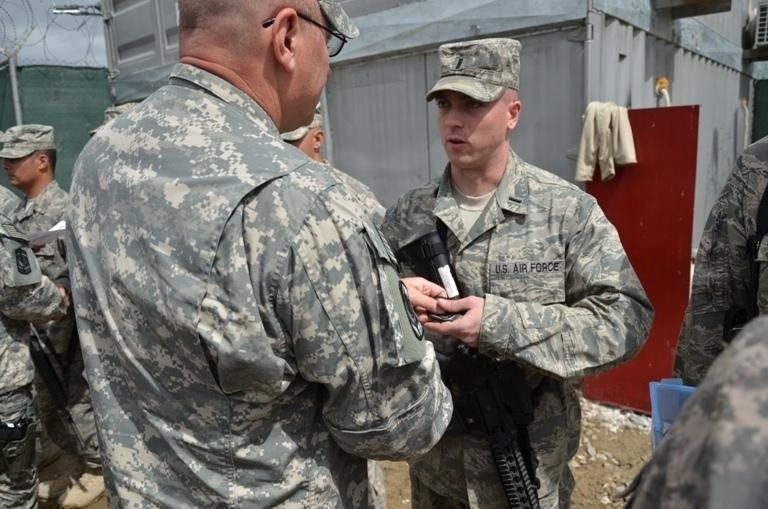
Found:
[39,462,106,508]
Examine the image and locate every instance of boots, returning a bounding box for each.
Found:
[35,423,62,471]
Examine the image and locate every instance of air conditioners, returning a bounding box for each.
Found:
[755,3,768,45]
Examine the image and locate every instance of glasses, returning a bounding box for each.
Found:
[262,11,347,57]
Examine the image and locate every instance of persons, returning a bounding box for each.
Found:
[63,0,454,509]
[376,38,655,509]
[281,98,385,509]
[676,136,767,384]
[0,135,61,472]
[629,313,768,509]
[0,227,73,509]
[1,122,105,508]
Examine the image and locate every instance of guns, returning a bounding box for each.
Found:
[0,417,32,446]
[397,231,542,509]
[30,334,86,450]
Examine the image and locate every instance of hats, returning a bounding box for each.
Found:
[0,124,56,158]
[427,38,521,101]
[320,0,360,38]
[280,100,321,140]
